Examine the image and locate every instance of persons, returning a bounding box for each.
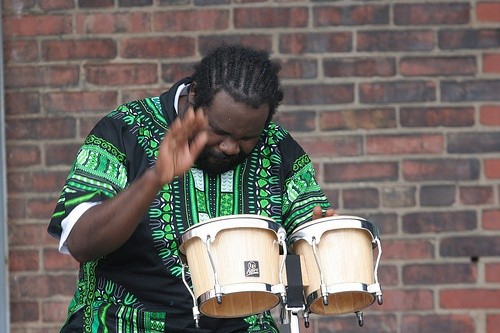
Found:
[47,45,339,333]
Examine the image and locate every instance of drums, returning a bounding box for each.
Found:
[288,216,383,328]
[177,214,287,329]
